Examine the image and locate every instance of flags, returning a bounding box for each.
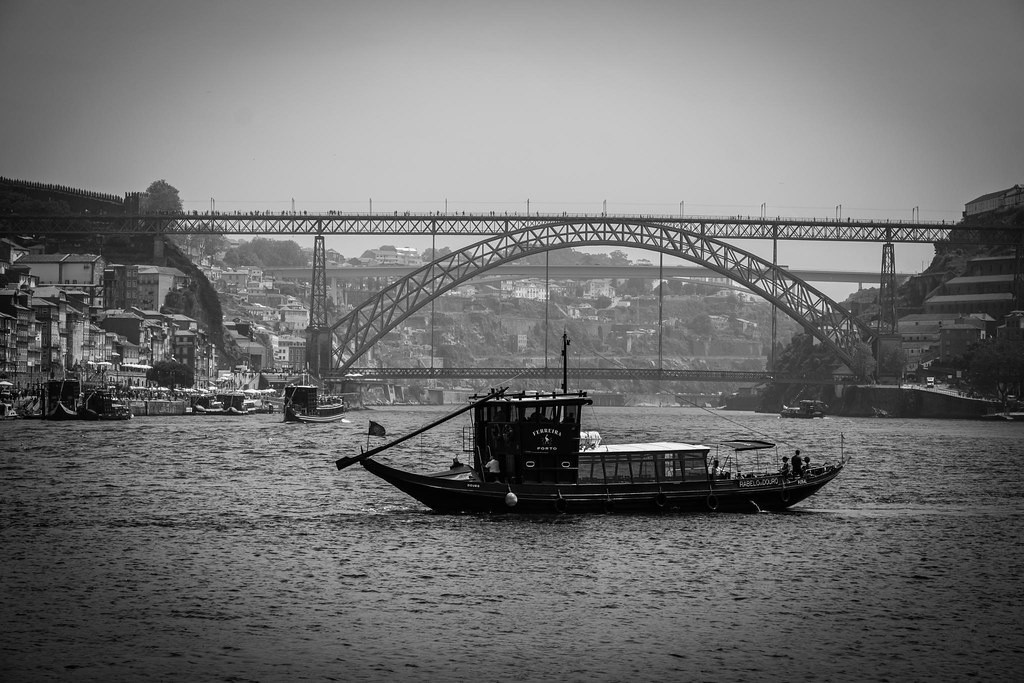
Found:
[368,420,385,437]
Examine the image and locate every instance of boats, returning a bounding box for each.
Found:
[779,399,826,419]
[336,325,851,514]
[2,366,133,420]
[284,382,347,423]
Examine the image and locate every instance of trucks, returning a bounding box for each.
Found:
[926,376,935,388]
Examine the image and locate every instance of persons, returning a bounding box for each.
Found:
[791,450,803,480]
[781,457,790,482]
[493,411,506,422]
[565,413,575,423]
[485,456,500,482]
[450,458,464,470]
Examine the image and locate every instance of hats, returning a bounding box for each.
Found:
[782,457,789,459]
